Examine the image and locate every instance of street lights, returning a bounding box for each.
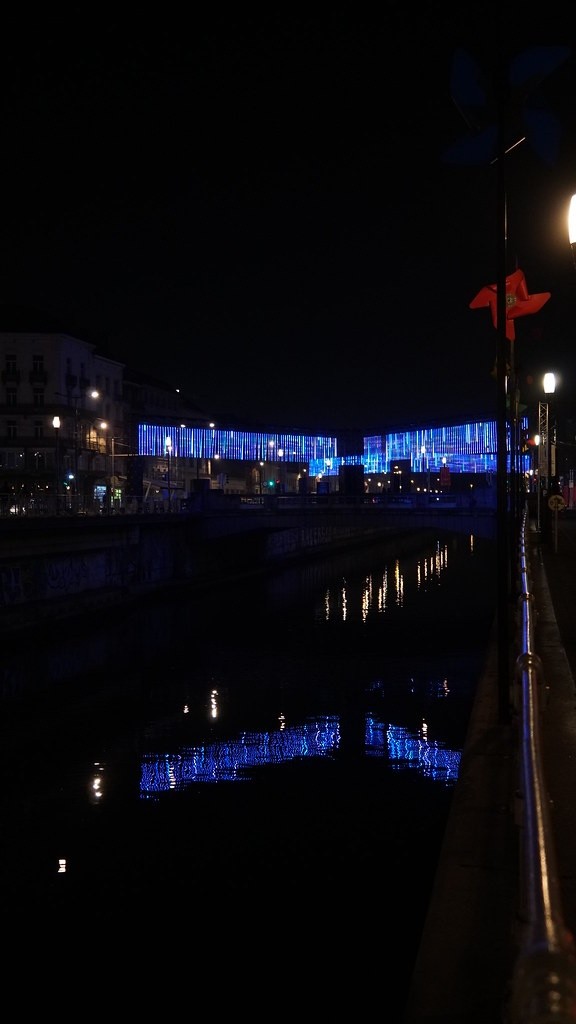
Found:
[211,455,219,481]
[442,457,446,467]
[260,461,264,494]
[543,373,556,530]
[303,469,306,499]
[52,417,61,516]
[166,437,171,512]
[421,445,425,472]
